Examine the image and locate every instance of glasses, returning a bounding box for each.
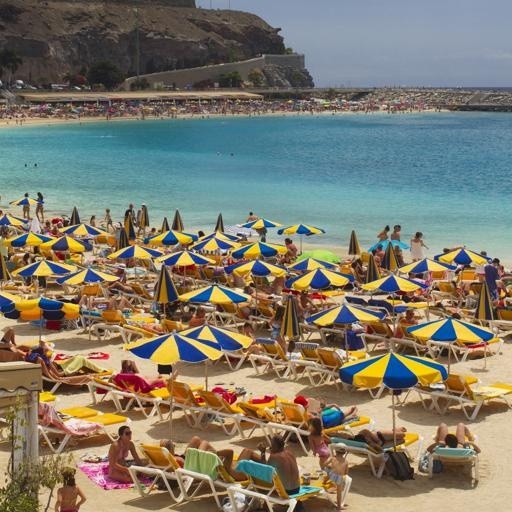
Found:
[125,431,132,436]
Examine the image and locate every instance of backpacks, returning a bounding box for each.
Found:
[385,450,415,480]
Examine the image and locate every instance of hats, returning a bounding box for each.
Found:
[116,264,126,270]
[294,396,310,408]
[335,443,347,452]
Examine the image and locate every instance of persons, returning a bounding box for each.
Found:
[0,192,511,512]
[0,91,511,127]
